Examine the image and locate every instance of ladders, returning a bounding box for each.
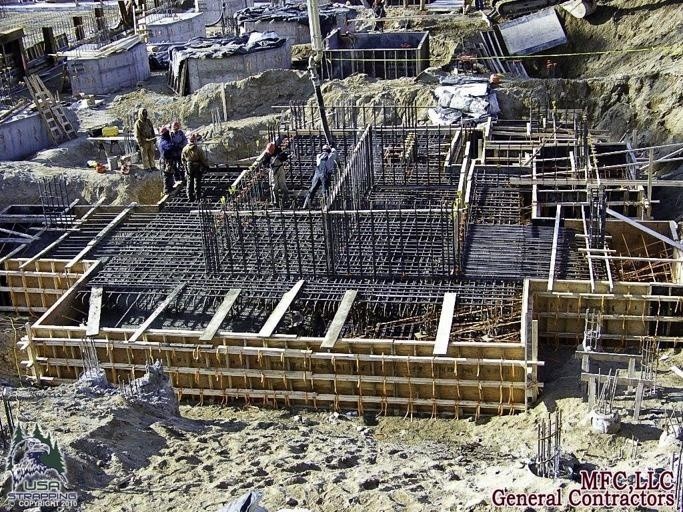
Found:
[23,72,78,147]
[133,4,147,43]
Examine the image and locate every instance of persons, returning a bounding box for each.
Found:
[134,108,159,172]
[158,127,175,195]
[262,143,289,207]
[302,145,337,210]
[181,134,205,202]
[170,122,187,181]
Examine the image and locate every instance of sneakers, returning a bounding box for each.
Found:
[152,167,159,171]
[145,167,152,172]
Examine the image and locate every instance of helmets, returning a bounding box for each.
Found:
[266,144,275,153]
[172,121,180,130]
[187,133,196,142]
[322,145,331,152]
[159,127,168,135]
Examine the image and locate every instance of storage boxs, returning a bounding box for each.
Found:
[102,126,118,136]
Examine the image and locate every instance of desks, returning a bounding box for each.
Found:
[86,136,144,160]
